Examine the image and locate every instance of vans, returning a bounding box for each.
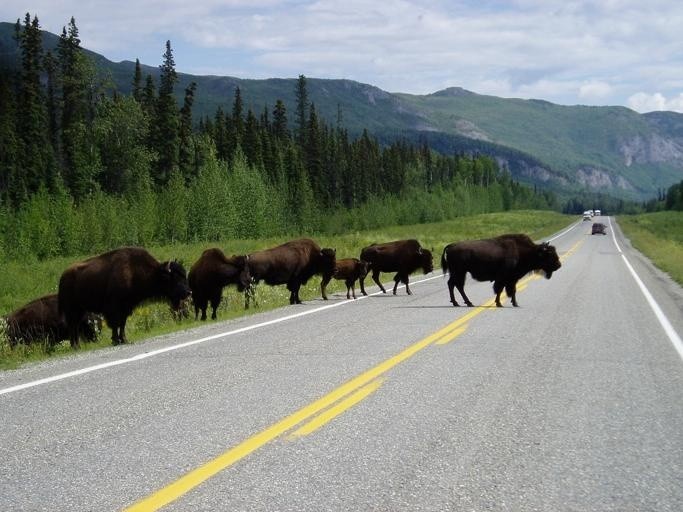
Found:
[592,223,607,235]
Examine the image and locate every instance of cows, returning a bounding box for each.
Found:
[440,233,561,307]
[320,259,372,300]
[360,239,432,295]
[245,239,338,309]
[57,247,192,350]
[2,294,103,358]
[187,248,251,320]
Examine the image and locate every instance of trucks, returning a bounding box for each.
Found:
[583,209,601,221]
